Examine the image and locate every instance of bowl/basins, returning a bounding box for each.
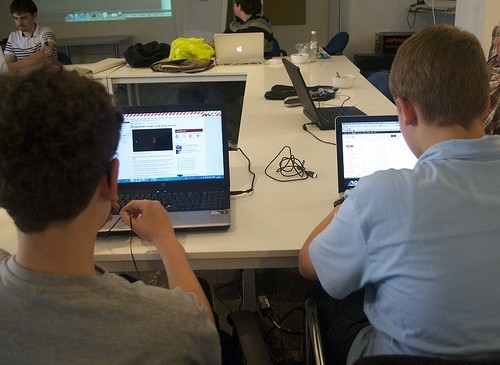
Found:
[332,74,355,88]
[290,54,308,63]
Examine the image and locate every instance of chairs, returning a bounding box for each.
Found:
[299,296,500,365]
[322,32,350,55]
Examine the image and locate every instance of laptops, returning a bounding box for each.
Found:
[94,104,230,235]
[214,32,264,64]
[335,115,417,196]
[282,58,368,129]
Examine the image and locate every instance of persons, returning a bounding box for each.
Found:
[0,0,58,69]
[209,0,273,58]
[0,70,223,365]
[298,25,500,365]
[365,25,500,136]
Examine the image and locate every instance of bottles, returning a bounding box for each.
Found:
[44,47,53,66]
[309,30,319,62]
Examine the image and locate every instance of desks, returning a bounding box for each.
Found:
[54,35,398,312]
[56,35,131,58]
[353,52,396,73]
